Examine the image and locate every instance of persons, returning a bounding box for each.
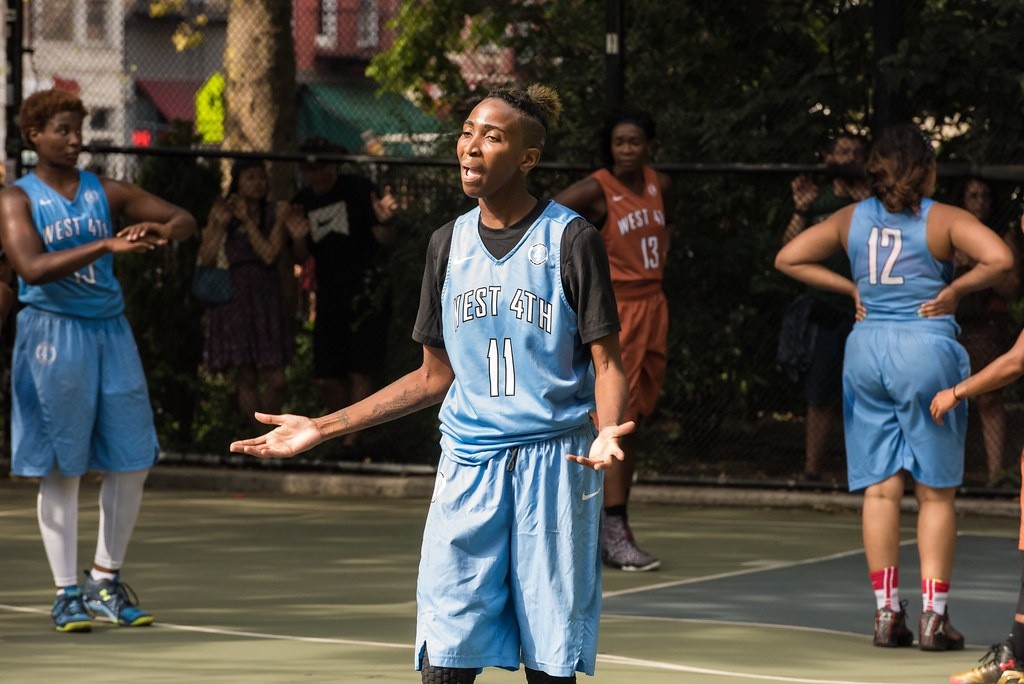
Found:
[928,324,1024,683]
[550,102,670,571]
[2,89,200,630]
[774,123,1016,652]
[228,79,637,684]
[1,132,1021,498]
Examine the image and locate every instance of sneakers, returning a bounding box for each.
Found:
[81,569,154,625]
[603,516,660,571]
[918,609,966,651]
[951,640,1024,684]
[50,593,92,632]
[873,604,915,648]
